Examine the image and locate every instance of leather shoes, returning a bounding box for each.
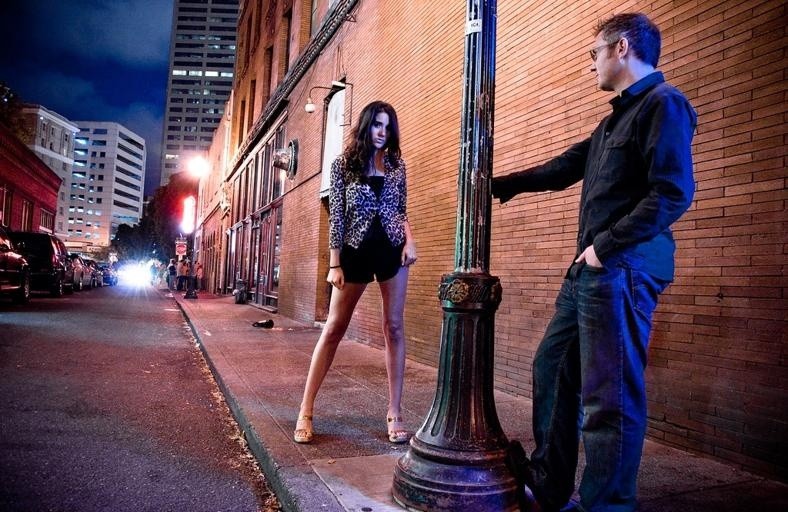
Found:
[507,438,559,509]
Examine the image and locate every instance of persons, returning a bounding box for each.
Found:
[291,100,418,445]
[489,9,703,511]
[149,257,204,292]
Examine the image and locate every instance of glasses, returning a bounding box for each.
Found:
[589,40,619,61]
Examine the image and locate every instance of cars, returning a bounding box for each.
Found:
[0,228,119,301]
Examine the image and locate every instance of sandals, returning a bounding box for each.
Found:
[387,411,409,443]
[293,410,314,443]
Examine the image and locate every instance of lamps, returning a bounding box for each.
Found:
[305,86,340,113]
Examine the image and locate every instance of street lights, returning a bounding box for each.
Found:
[183,154,209,300]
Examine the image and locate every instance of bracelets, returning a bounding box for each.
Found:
[329,265,341,269]
